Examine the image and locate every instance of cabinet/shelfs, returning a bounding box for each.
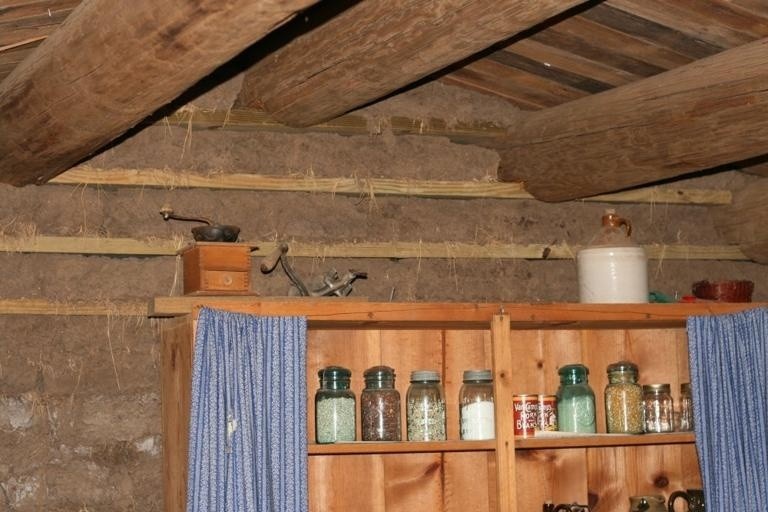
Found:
[147,296,768,512]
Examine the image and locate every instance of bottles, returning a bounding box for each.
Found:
[556,364,596,434]
[406,369,447,442]
[603,360,642,435]
[314,365,357,445]
[361,365,403,443]
[458,368,495,441]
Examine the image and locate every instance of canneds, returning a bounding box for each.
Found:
[512,393,538,435]
[537,394,559,431]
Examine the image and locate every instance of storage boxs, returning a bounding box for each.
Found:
[177,241,260,296]
